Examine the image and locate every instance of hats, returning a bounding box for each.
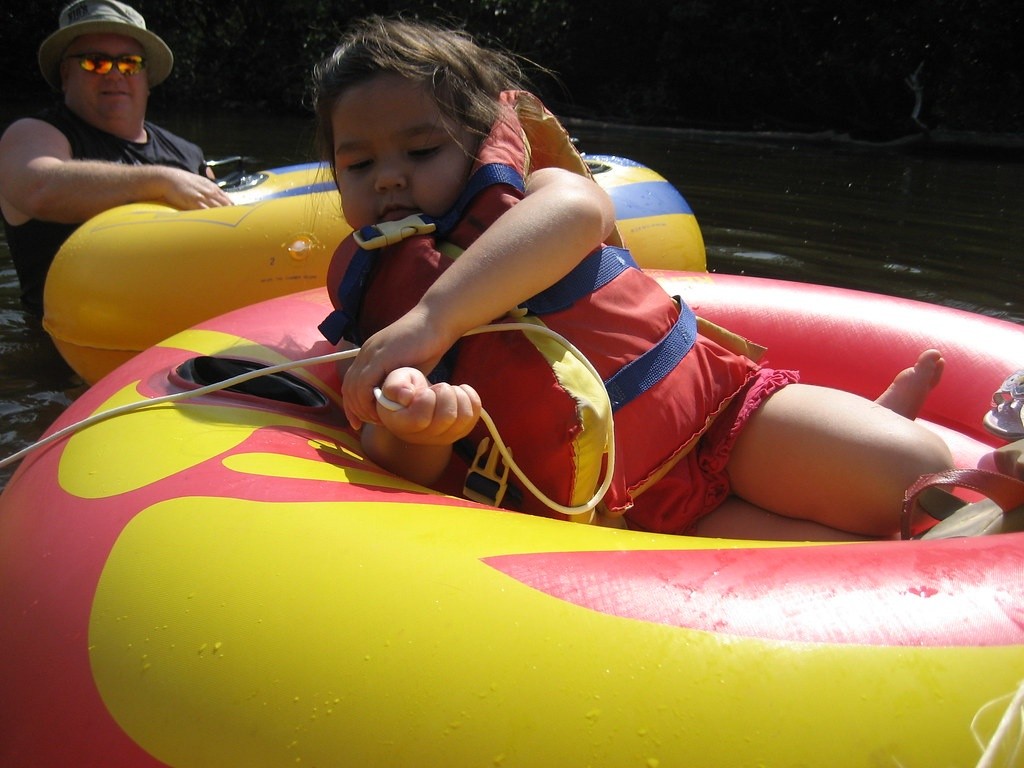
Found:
[38,0,174,92]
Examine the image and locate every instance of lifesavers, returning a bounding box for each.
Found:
[0,269,1024,768]
[38,152,713,394]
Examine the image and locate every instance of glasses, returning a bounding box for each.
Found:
[65,52,147,76]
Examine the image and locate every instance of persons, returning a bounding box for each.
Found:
[313,19,955,544]
[0,0,237,317]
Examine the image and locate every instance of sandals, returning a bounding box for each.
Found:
[901,439,1024,540]
[983,371,1024,439]
